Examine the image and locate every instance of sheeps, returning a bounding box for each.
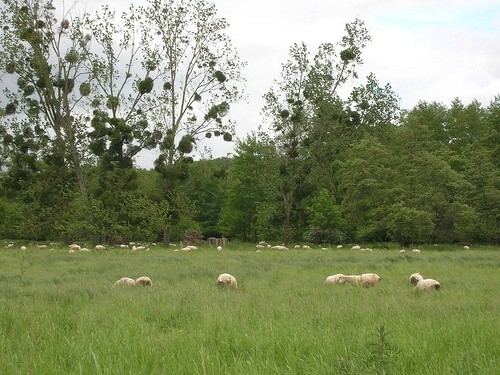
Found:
[408,272,441,292]
[112,276,153,288]
[325,272,381,287]
[2,239,471,253]
[216,273,238,288]
